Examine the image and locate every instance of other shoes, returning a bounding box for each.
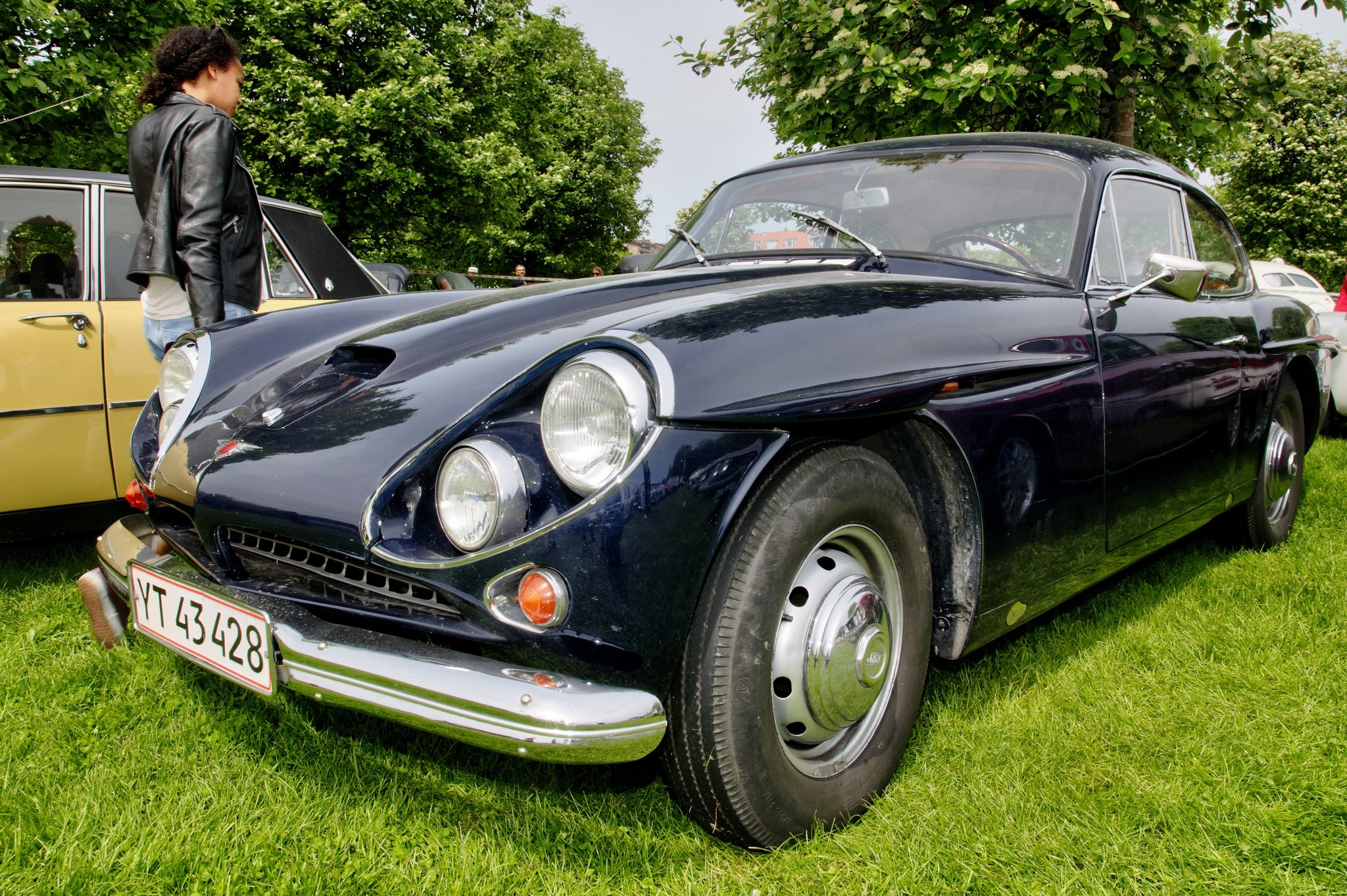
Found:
[77,565,134,651]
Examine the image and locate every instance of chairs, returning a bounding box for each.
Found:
[29,252,69,299]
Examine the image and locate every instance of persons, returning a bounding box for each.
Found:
[80,25,264,652]
[432,265,603,292]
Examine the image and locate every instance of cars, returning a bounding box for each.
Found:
[0,164,391,516]
[75,134,1331,857]
[1246,258,1347,433]
